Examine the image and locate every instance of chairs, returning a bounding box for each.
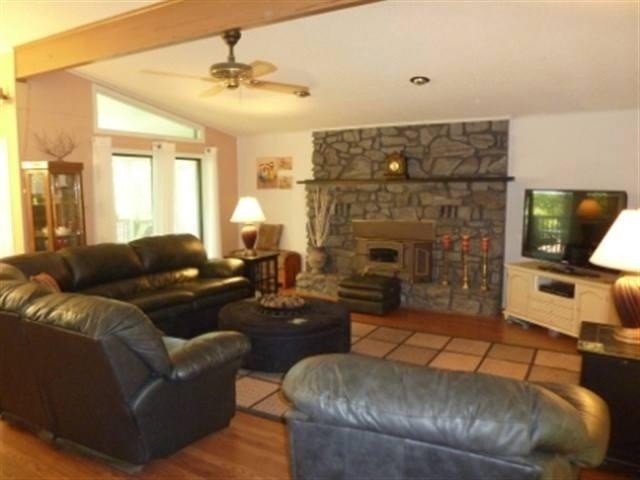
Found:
[277,353,613,479]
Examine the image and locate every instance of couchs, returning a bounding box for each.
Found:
[1,234,259,478]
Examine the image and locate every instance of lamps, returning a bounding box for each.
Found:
[224,192,266,254]
[585,203,639,343]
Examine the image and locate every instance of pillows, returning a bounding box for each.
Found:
[28,263,62,295]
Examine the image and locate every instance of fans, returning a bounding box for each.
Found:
[142,26,315,104]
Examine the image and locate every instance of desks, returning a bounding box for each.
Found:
[222,248,281,298]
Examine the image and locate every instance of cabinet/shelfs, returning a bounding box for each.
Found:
[21,158,89,253]
[572,317,639,478]
[499,257,620,339]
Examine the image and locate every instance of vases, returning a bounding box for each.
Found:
[307,246,328,273]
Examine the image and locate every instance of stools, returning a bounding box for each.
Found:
[336,270,402,317]
[213,292,353,375]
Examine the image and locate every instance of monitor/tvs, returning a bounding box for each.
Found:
[521,189,628,278]
